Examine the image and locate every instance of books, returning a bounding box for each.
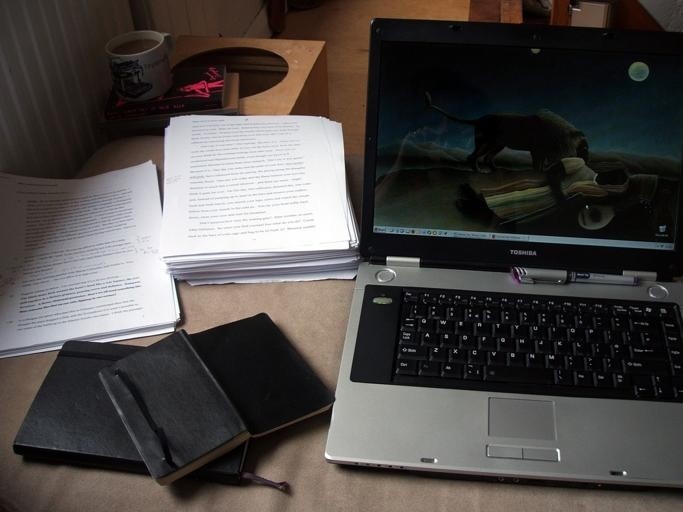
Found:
[105,63,241,137]
[12,340,258,487]
[99,312,337,488]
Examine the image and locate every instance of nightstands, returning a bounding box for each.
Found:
[170,34,329,118]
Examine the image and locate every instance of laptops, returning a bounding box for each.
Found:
[324,17,683,491]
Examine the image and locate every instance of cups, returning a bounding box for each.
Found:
[104,29,175,101]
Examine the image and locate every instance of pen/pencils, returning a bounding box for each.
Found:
[512,265,639,287]
